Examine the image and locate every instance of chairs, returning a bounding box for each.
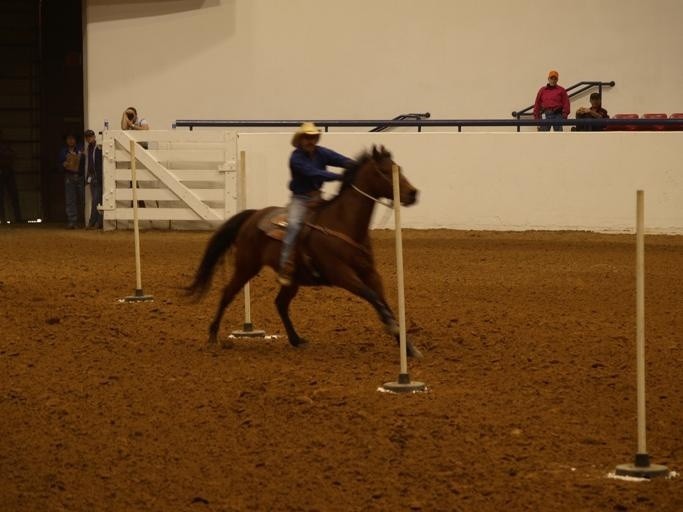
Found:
[606,112,683,130]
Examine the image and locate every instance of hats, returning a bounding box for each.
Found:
[549,70,559,79]
[590,93,600,100]
[83,130,94,136]
[290,122,322,149]
[121,110,135,130]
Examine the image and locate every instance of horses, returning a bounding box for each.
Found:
[175,142,419,360]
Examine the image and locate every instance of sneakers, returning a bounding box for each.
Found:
[277,270,292,287]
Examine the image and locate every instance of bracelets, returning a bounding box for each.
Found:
[130,124,135,128]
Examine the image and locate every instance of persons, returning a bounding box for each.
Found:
[532,71,570,131]
[0,131,24,225]
[83,130,102,231]
[57,132,85,229]
[121,107,150,150]
[274,121,356,287]
[571,92,609,131]
